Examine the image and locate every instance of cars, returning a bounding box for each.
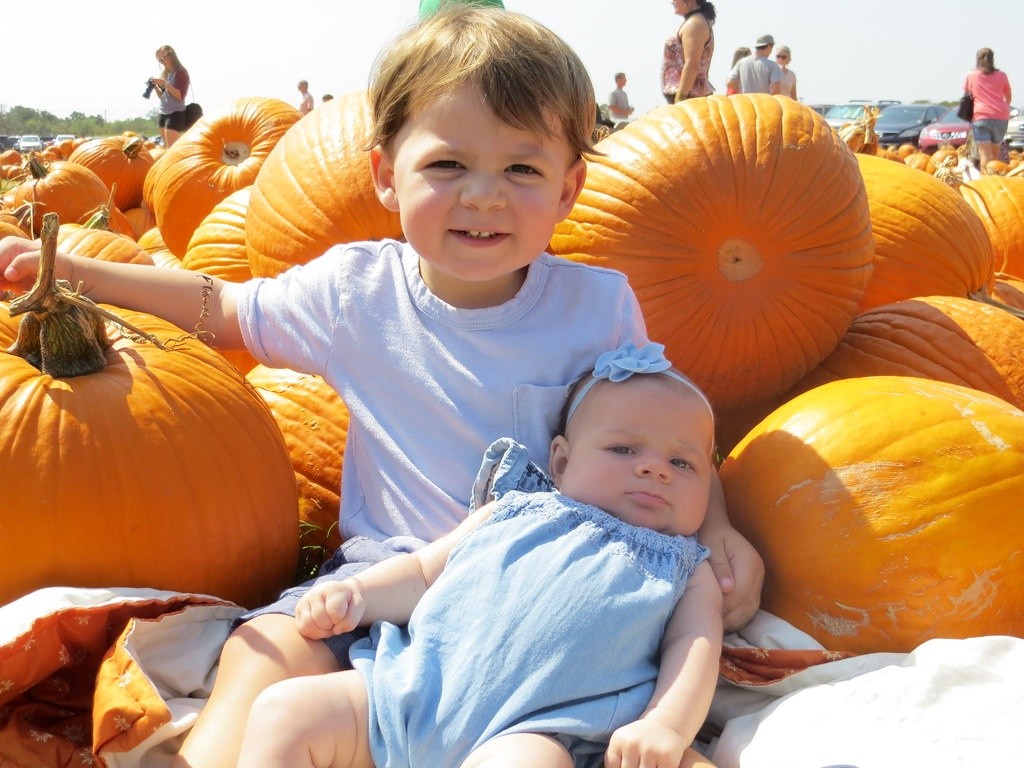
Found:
[52,133,76,148]
[865,104,950,149]
[149,135,165,148]
[997,107,1024,152]
[822,99,903,135]
[0,135,56,155]
[917,105,1021,157]
[805,104,835,117]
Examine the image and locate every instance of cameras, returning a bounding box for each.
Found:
[142,79,156,99]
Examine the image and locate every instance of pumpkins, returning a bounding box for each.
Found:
[0,90,1024,656]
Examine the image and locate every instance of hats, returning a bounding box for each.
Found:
[755,34,774,47]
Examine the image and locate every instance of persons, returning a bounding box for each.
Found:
[607,73,634,121]
[146,46,190,149]
[238,341,724,768]
[726,34,798,102]
[962,48,1012,171]
[660,0,716,105]
[297,80,314,116]
[0,8,763,768]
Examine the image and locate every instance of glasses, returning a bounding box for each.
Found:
[159,52,168,63]
[777,55,788,59]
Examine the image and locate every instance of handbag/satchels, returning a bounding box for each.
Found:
[185,103,202,129]
[958,95,973,122]
